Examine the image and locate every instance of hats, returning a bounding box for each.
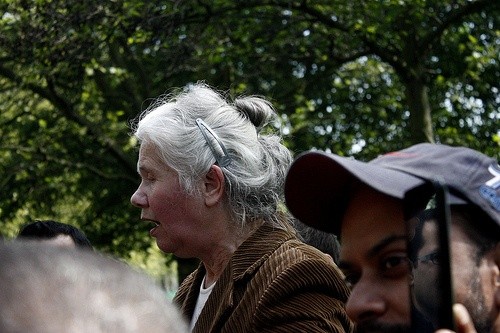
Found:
[283,142,500,242]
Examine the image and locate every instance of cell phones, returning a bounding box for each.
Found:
[403,180,454,333]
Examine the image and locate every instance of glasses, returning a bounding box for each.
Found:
[411,249,438,268]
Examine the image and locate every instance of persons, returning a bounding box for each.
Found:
[284,140,500,333]
[0,218,189,332]
[128,76,360,333]
